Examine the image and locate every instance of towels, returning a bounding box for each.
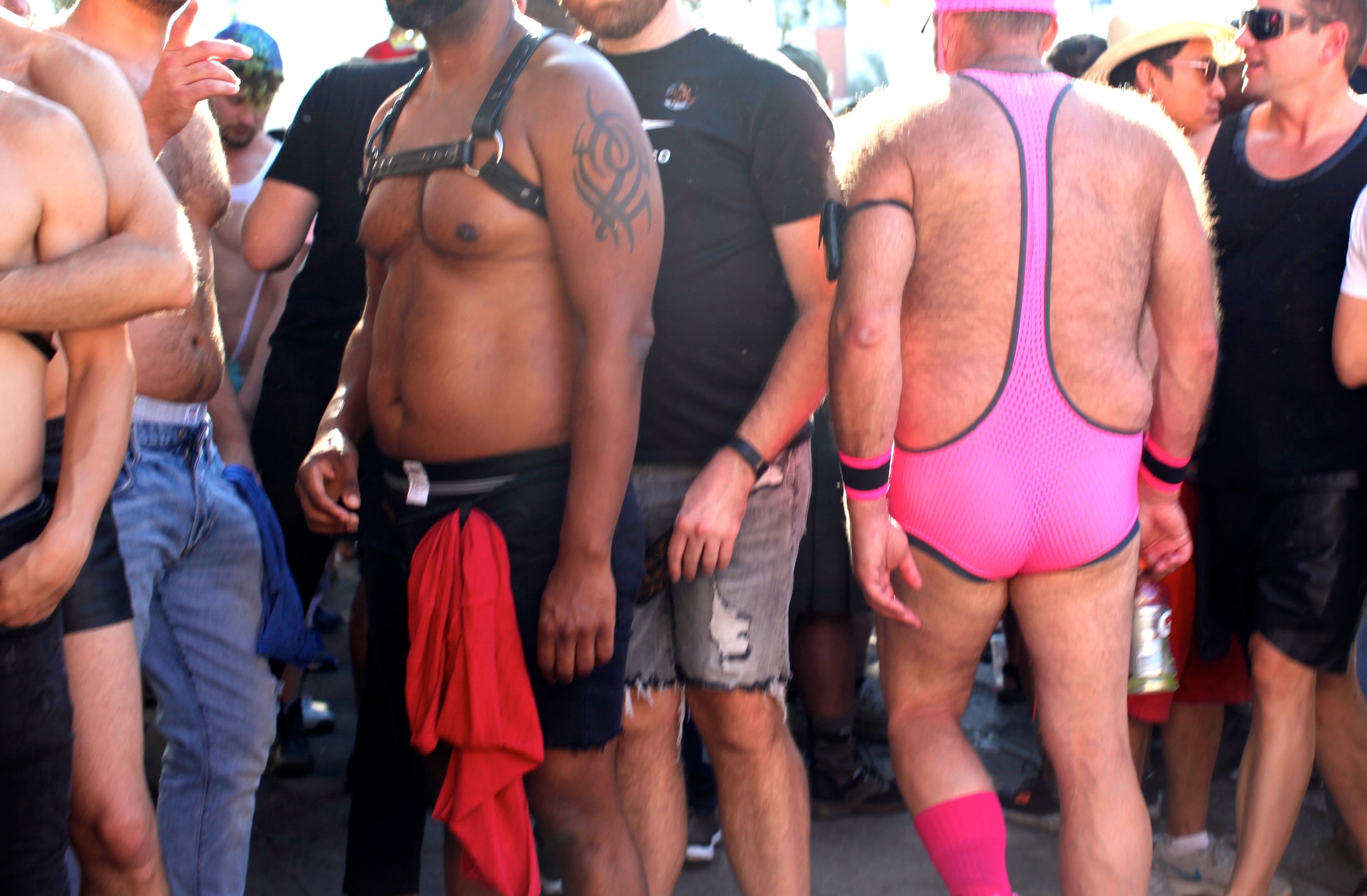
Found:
[216,461,328,670]
[400,505,549,896]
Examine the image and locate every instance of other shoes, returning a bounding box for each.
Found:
[531,827,564,893]
[998,778,1065,831]
[270,697,314,774]
[684,807,724,863]
[316,610,341,632]
[301,693,339,734]
[309,647,336,668]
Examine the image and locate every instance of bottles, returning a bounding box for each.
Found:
[1126,556,1179,694]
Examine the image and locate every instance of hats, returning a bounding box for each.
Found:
[1083,9,1243,88]
[214,21,284,80]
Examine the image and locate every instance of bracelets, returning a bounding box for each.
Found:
[1140,428,1190,492]
[726,431,770,484]
[840,447,890,501]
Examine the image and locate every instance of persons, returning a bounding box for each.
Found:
[0,0,1366,896]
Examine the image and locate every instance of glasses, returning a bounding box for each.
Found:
[1151,58,1218,82]
[1238,7,1344,42]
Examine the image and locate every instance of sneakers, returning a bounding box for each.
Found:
[811,754,905,817]
[1153,837,1293,896]
